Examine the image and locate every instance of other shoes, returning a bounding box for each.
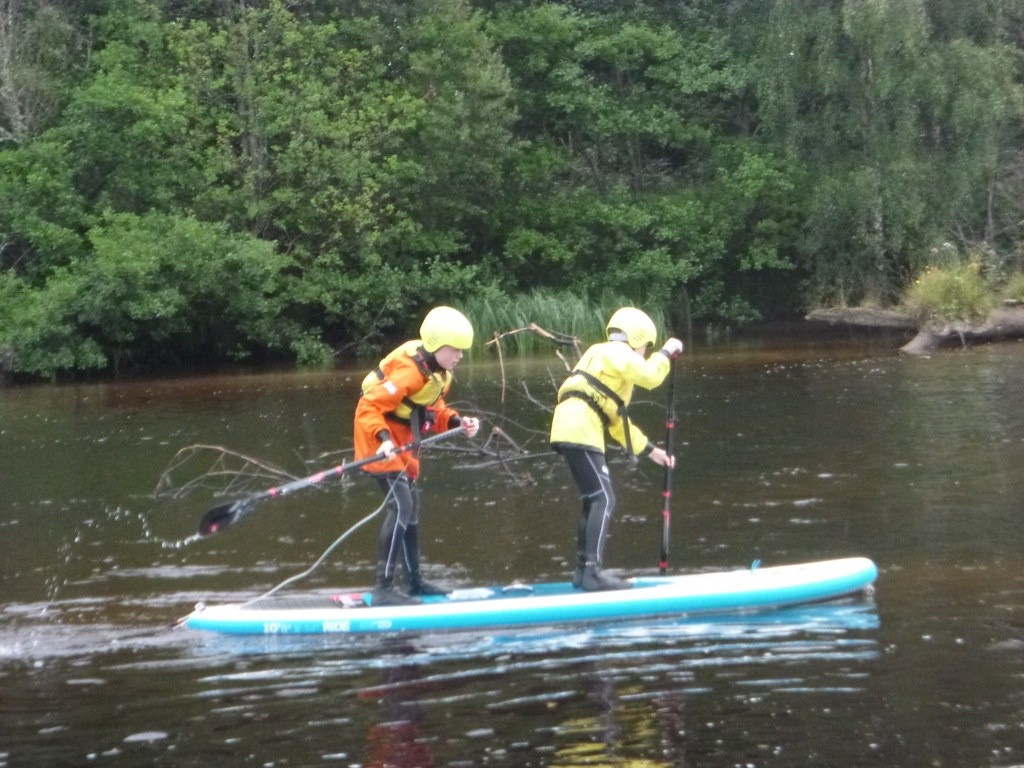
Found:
[371,579,421,606]
[572,565,633,591]
[410,577,454,595]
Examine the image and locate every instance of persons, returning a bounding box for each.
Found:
[354,306,479,606]
[549,307,683,591]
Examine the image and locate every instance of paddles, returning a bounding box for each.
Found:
[198,418,475,538]
[659,347,682,576]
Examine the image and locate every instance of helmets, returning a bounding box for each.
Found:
[420,305,473,353]
[605,307,657,348]
